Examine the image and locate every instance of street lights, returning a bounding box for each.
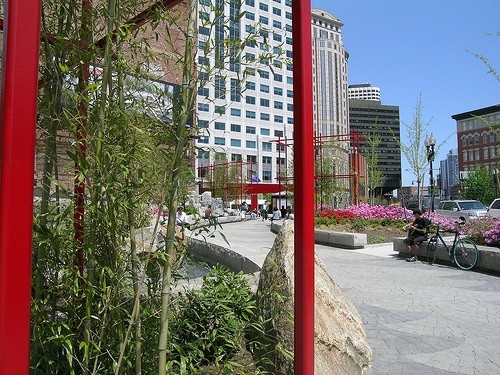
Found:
[424,132,436,213]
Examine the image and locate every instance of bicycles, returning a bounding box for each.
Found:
[426,220,479,271]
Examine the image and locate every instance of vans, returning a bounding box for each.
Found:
[438,200,490,224]
[485,198,500,220]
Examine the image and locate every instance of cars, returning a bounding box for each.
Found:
[412,189,438,203]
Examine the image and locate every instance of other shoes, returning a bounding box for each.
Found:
[407,256,418,262]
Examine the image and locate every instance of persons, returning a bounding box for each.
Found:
[279,206,287,218]
[235,209,240,216]
[240,201,250,221]
[287,207,292,214]
[258,209,268,222]
[269,207,281,221]
[251,208,258,220]
[359,200,362,205]
[176,207,190,230]
[402,209,429,262]
[205,205,212,218]
